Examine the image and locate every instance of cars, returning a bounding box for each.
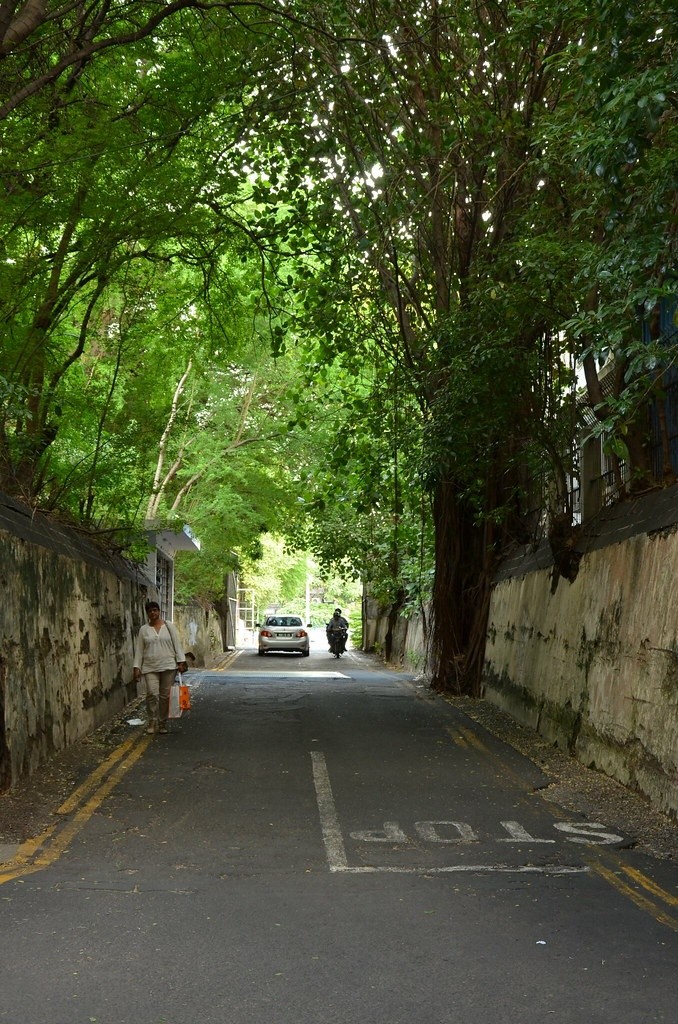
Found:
[255,615,313,656]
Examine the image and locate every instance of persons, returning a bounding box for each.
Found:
[132,601,186,734]
[327,609,349,653]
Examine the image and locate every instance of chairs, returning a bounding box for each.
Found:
[269,620,278,625]
[290,619,300,626]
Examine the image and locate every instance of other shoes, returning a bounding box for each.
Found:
[159,726,167,732]
[147,727,154,733]
[344,648,347,651]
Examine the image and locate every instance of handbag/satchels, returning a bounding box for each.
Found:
[168,672,191,718]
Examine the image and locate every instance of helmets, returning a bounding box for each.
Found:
[333,611,340,618]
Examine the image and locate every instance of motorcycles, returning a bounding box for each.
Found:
[325,624,348,658]
